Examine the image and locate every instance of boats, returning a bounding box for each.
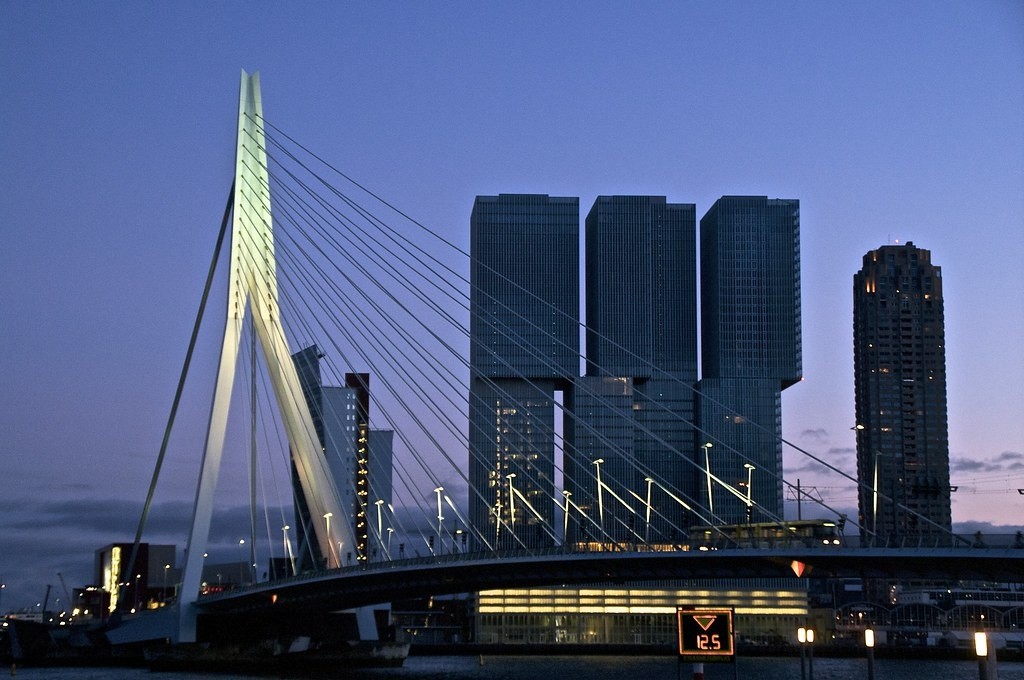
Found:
[141,599,413,674]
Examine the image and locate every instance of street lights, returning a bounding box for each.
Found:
[433,486,444,554]
[806,629,816,680]
[506,472,517,549]
[592,458,605,543]
[645,477,655,543]
[562,490,572,542]
[701,441,714,523]
[374,499,384,563]
[743,464,756,523]
[796,627,806,680]
[863,628,876,680]
[323,512,333,569]
[972,631,998,680]
[386,528,394,562]
[133,538,246,614]
[280,526,290,582]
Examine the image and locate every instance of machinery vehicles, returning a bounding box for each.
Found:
[42,583,54,615]
